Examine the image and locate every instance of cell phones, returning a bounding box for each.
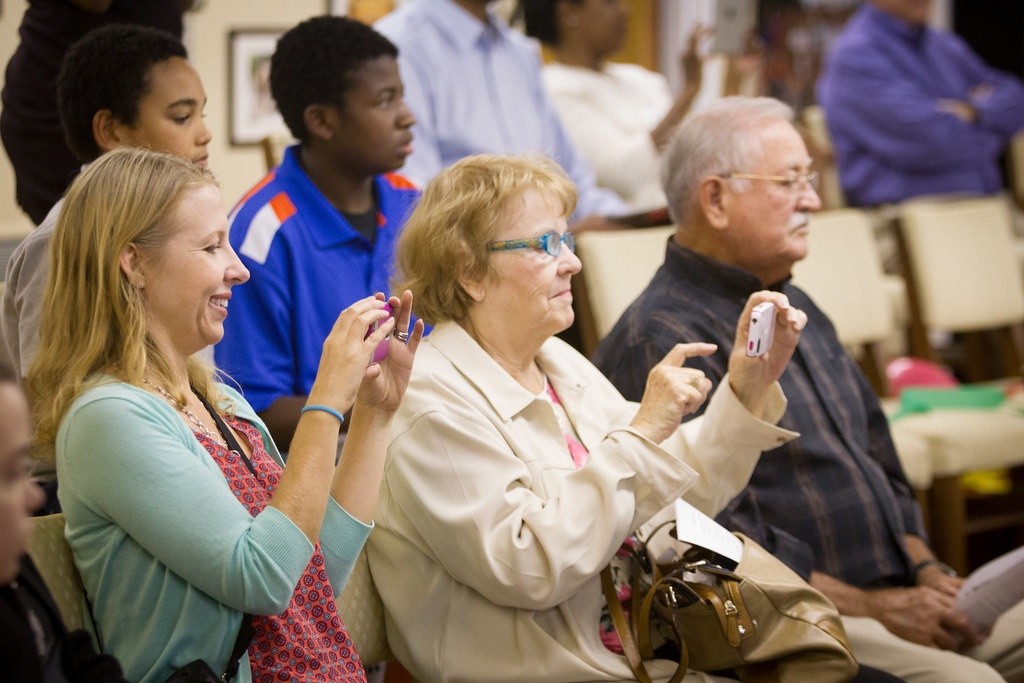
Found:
[746,301,777,356]
[710,1,756,54]
[367,300,393,362]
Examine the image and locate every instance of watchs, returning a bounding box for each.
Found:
[908,559,958,587]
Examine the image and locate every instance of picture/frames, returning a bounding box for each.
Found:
[225,27,291,146]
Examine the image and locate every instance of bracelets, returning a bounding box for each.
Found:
[300,405,344,424]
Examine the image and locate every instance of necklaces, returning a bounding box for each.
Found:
[101,357,242,459]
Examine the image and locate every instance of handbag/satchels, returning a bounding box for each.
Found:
[602,541,854,683]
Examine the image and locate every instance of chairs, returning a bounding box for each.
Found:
[17,513,102,658]
[790,206,886,351]
[884,189,1024,572]
[571,225,676,356]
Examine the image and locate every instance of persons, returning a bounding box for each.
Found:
[363,0,628,228]
[814,0,1024,209]
[0,144,425,683]
[362,151,808,683]
[587,94,1024,683]
[520,0,720,231]
[745,0,813,103]
[213,15,434,453]
[0,25,211,384]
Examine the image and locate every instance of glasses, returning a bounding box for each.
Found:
[486,228,577,259]
[724,169,823,201]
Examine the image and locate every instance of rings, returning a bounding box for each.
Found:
[393,330,409,342]
